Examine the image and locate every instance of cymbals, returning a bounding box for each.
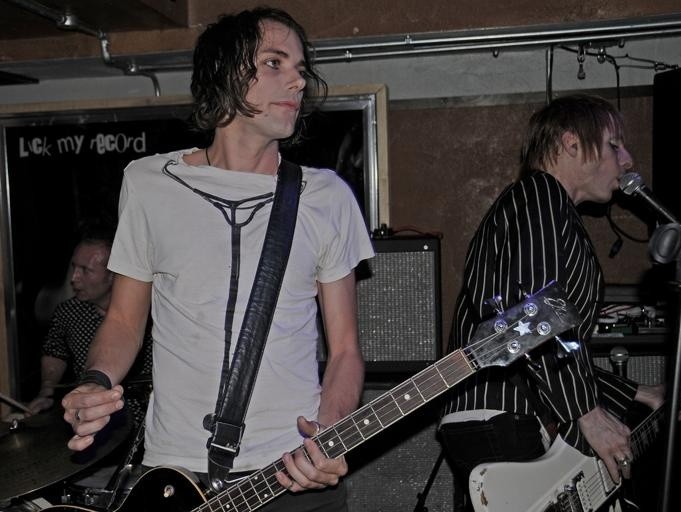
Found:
[0,402,134,504]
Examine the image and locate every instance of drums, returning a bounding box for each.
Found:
[47,447,132,511]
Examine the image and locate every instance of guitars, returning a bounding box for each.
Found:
[44,280,581,512]
[467,400,670,512]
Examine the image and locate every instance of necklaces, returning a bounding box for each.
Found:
[202,145,211,167]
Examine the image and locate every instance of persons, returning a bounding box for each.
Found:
[437,94,680,511]
[57,7,377,512]
[24,236,152,429]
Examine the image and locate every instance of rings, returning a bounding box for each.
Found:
[312,420,320,434]
[617,455,630,466]
[287,480,294,490]
[325,473,330,485]
[74,408,81,423]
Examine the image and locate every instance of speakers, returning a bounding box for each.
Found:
[316,233,441,382]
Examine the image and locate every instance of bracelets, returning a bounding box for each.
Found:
[77,370,112,390]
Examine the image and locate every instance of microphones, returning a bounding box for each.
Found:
[620,172,681,225]
[610,346,628,379]
